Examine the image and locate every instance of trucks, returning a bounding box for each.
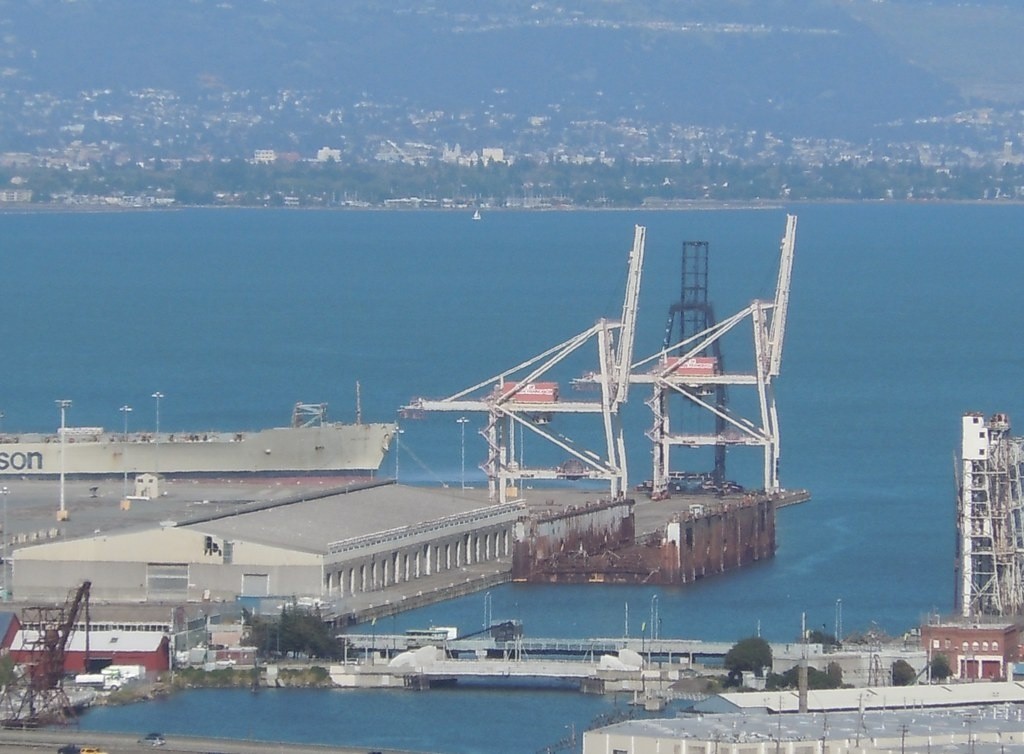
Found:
[75,674,123,691]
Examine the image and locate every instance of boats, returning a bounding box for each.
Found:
[0,402,399,482]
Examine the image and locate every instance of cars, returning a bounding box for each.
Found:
[58,744,80,754]
[80,747,107,754]
[216,658,237,666]
[340,658,359,666]
[137,737,166,747]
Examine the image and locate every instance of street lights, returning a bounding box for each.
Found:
[0,487,11,599]
[456,416,469,490]
[372,618,376,666]
[119,406,133,511]
[152,392,163,475]
[935,615,940,625]
[485,592,490,630]
[835,599,841,640]
[392,427,405,485]
[55,400,72,521]
[650,595,657,639]
[641,622,646,692]
